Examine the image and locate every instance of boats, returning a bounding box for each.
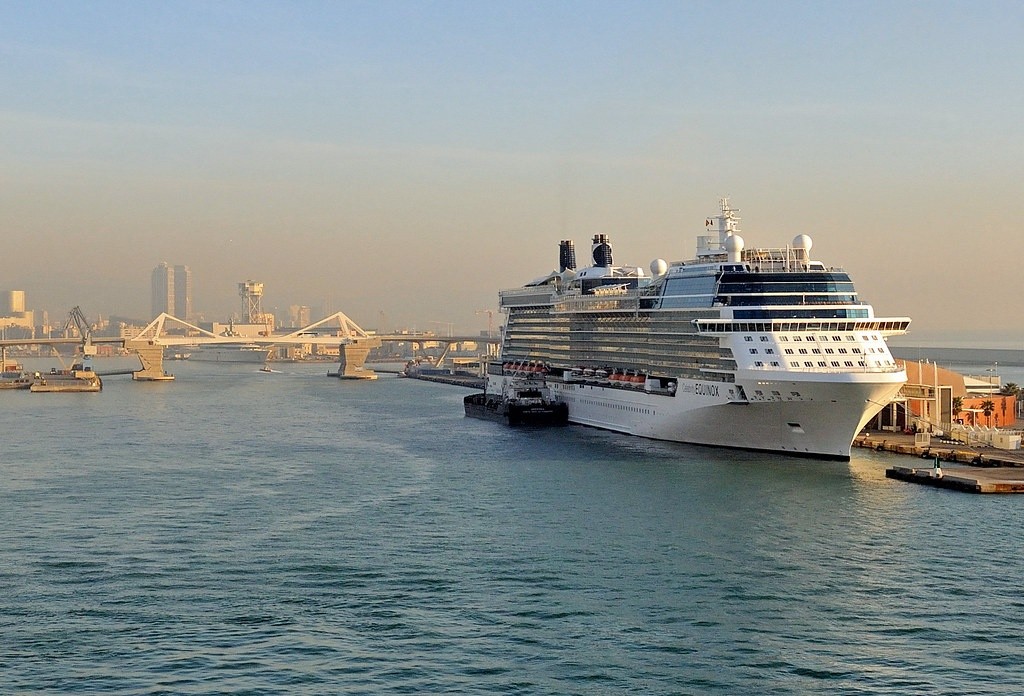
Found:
[163,353,191,360]
[259,368,271,372]
[463,349,569,430]
[0,379,34,389]
[84,356,92,360]
[119,350,128,356]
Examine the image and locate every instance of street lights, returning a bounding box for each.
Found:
[474,311,492,339]
[985,368,995,442]
[991,360,999,377]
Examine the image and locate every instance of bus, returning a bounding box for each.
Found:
[297,332,319,338]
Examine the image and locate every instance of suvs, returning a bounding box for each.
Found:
[41,377,47,386]
[50,368,56,374]
[34,371,40,379]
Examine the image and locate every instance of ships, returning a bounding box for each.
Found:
[189,345,271,363]
[485,196,912,463]
